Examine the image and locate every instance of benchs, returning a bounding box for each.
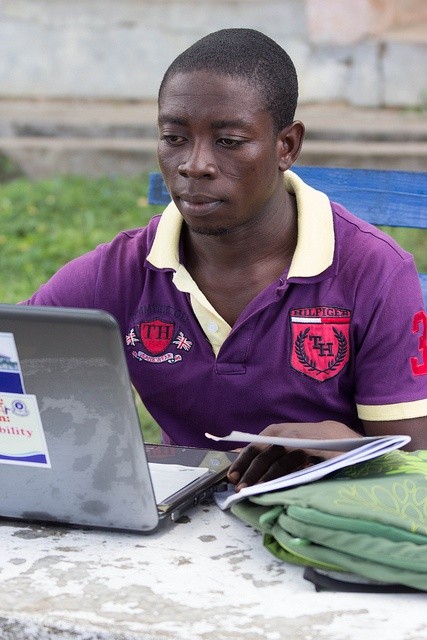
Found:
[149,164,427,317]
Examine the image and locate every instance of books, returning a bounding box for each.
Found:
[202,430,411,510]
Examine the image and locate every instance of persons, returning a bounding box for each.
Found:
[12,29,427,493]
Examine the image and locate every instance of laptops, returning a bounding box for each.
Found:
[1,303,243,534]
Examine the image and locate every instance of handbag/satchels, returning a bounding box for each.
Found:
[231,449,427,595]
